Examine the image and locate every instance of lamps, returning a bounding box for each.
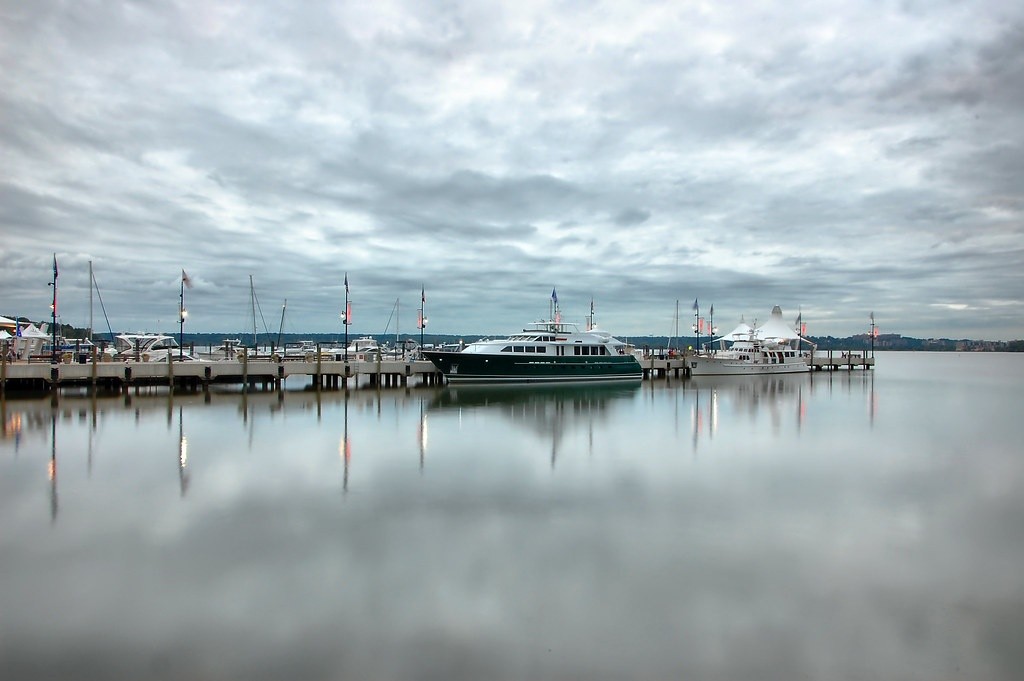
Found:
[692,324,697,330]
[182,308,188,320]
[423,317,428,323]
[341,311,346,318]
[867,331,872,336]
[795,328,800,333]
[712,327,718,333]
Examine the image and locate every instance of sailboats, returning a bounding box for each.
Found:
[14,338,102,359]
[333,295,434,361]
[252,300,344,360]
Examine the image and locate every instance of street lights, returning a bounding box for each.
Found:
[342,287,349,364]
[48,254,61,364]
[178,281,188,361]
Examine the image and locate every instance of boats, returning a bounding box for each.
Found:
[197,339,254,361]
[115,332,201,361]
[418,287,644,385]
[686,319,810,375]
